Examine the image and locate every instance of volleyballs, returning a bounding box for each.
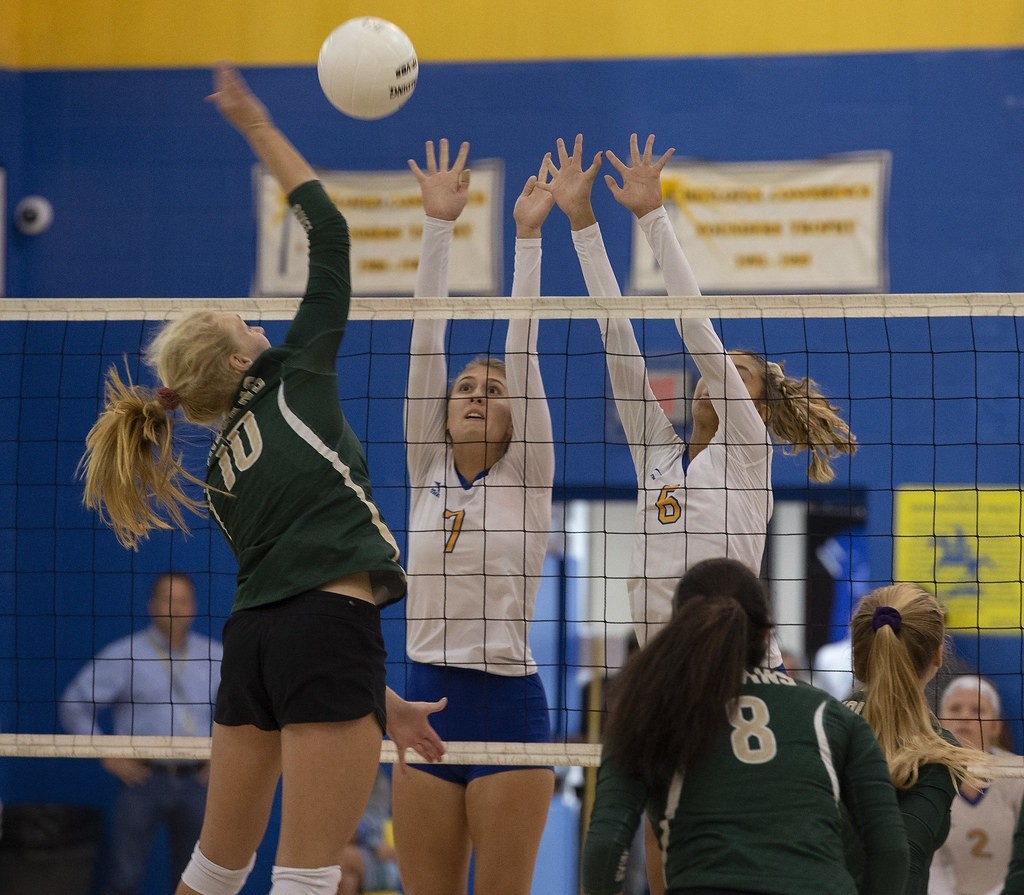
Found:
[317,15,419,120]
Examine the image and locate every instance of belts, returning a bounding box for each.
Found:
[246,597,382,634]
[142,764,205,776]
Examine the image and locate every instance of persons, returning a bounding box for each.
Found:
[927,671,1024,895]
[577,557,910,895]
[536,132,858,895]
[81,73,446,895]
[841,583,1001,895]
[58,571,224,895]
[334,771,388,895]
[389,137,554,895]
[583,628,654,894]
